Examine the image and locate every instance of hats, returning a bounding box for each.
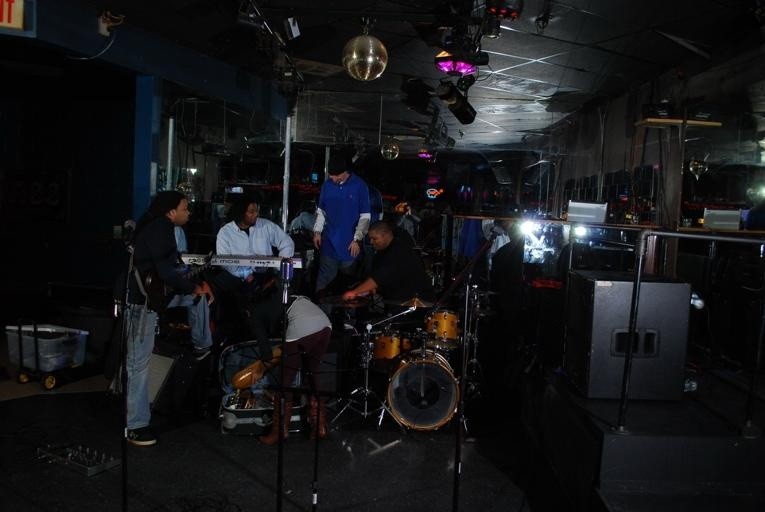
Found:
[328,156,345,175]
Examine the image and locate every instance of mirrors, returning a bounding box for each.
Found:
[674,78,765,369]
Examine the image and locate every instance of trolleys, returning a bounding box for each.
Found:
[16,317,90,392]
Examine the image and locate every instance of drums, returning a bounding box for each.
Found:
[382,349,462,431]
[369,331,420,372]
[426,310,460,350]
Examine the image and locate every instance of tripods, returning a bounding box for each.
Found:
[330,347,404,431]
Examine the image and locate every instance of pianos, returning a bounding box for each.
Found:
[180,252,303,271]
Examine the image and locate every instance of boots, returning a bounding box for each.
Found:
[258,395,292,446]
[306,395,330,442]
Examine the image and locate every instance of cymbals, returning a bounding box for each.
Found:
[394,298,434,307]
[320,296,368,307]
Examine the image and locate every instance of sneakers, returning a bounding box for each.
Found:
[126,425,158,447]
[192,340,215,360]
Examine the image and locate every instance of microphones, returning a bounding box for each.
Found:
[123,219,135,245]
[281,258,292,302]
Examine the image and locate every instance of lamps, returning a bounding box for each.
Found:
[341,16,388,82]
[401,73,477,125]
[433,3,522,75]
[380,134,400,161]
[282,8,301,40]
[424,115,455,152]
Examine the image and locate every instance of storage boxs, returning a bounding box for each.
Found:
[5,326,89,374]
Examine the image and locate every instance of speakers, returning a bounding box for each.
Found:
[593,435,765,511]
[567,268,694,401]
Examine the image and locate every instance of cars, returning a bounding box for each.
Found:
[520,191,746,225]
